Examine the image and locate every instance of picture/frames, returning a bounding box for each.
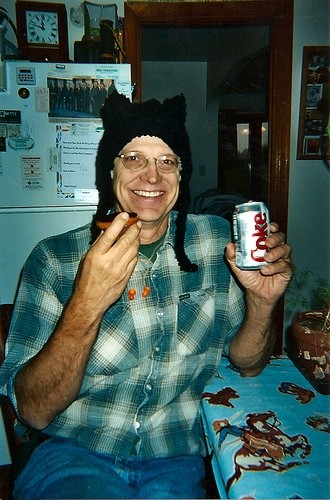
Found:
[297,47,330,159]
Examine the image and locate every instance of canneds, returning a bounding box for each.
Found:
[231,200,272,271]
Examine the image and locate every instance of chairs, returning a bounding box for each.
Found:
[0,304,46,473]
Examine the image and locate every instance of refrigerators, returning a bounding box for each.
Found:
[0,62,132,306]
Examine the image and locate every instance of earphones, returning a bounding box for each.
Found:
[128,289,136,300]
[142,286,151,297]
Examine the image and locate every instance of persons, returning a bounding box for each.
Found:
[0,90,294,500]
[47,80,114,119]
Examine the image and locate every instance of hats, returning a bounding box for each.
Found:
[89,88,201,274]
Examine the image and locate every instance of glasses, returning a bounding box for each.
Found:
[116,151,182,174]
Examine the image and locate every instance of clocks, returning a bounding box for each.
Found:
[16,0,68,59]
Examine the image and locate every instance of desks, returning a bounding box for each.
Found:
[198,357,330,499]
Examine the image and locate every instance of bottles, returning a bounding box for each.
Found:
[101,19,115,61]
[0,7,7,61]
